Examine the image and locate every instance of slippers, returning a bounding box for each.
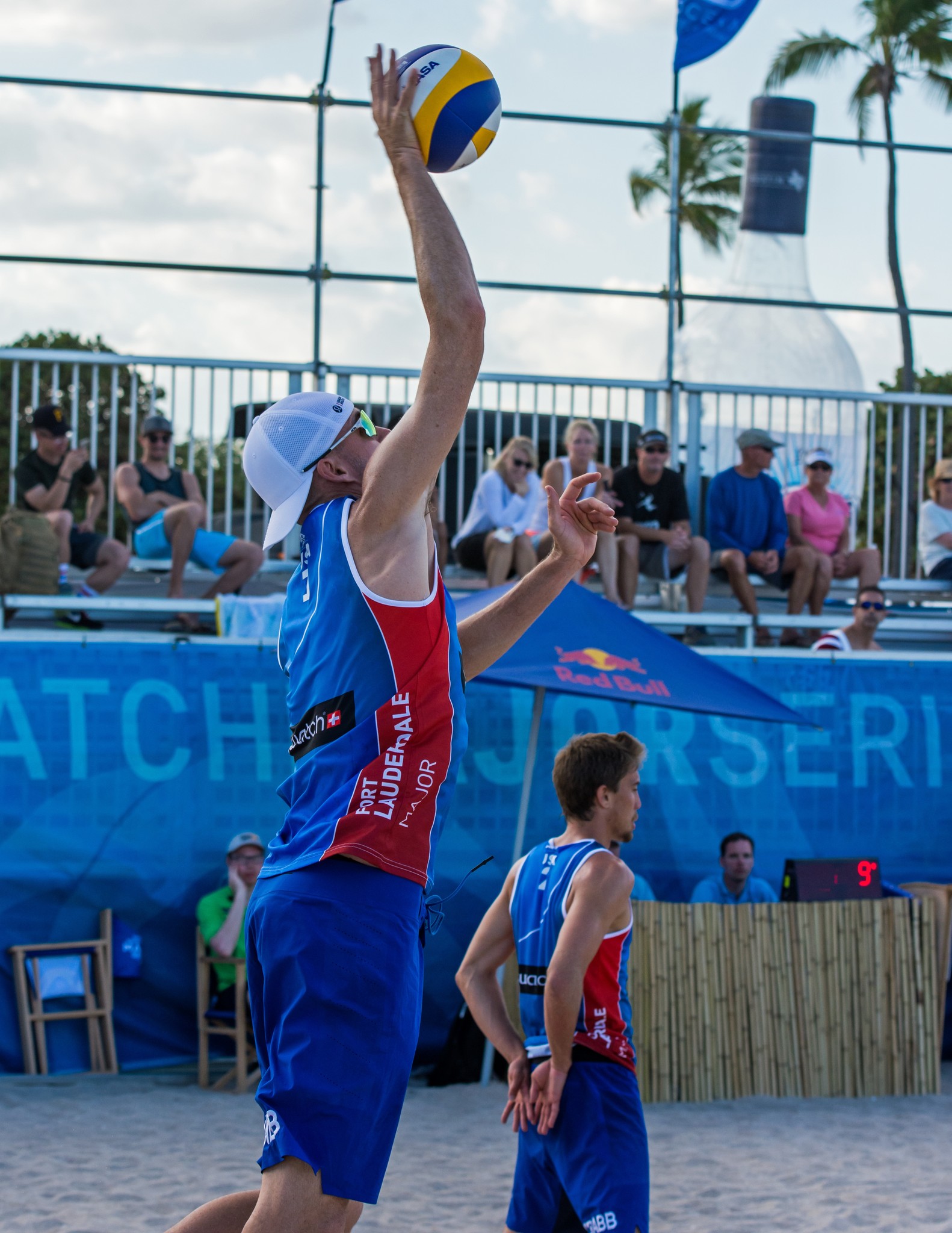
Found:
[756,634,774,646]
[779,634,810,648]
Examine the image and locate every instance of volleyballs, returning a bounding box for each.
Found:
[384,44,502,175]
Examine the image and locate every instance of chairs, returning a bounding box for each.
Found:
[196,925,261,1094]
[8,909,145,1075]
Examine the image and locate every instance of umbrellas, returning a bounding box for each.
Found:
[453,573,818,1088]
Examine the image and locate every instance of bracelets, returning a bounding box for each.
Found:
[59,475,71,483]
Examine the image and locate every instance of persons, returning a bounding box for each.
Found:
[114,412,265,633]
[196,832,265,1046]
[13,405,129,632]
[455,731,649,1233]
[609,839,658,902]
[689,834,781,905]
[452,417,886,655]
[917,458,952,582]
[165,45,618,1233]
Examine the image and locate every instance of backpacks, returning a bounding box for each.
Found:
[0,506,60,596]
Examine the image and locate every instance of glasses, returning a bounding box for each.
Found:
[860,601,884,611]
[513,458,534,470]
[761,445,773,453]
[644,446,667,454]
[938,478,952,483]
[39,428,74,439]
[301,409,378,472]
[807,463,831,471]
[147,434,171,444]
[233,854,264,866]
[573,439,590,445]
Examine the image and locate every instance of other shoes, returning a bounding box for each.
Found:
[65,608,104,630]
[683,627,715,645]
[163,612,210,634]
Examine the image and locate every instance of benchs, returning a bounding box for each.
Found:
[70,564,952,642]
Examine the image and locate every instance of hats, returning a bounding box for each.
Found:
[738,429,786,448]
[928,459,952,496]
[804,452,835,469]
[636,430,668,448]
[241,392,355,551]
[142,417,172,436]
[33,405,72,435]
